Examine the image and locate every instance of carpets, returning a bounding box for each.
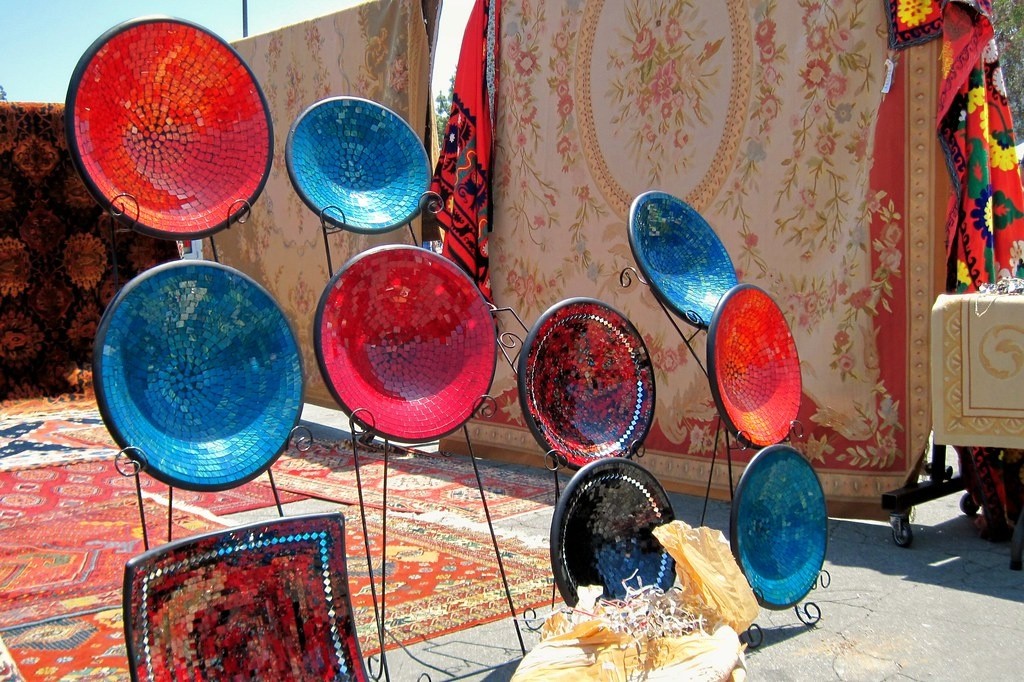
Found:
[0,406,567,682]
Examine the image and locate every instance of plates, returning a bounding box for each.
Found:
[314,244,497,443]
[626,190,738,329]
[518,297,658,470]
[549,458,677,605]
[93,259,305,484]
[122,512,370,681]
[706,283,803,448]
[283,95,433,235]
[729,444,828,610]
[68,17,275,240]
